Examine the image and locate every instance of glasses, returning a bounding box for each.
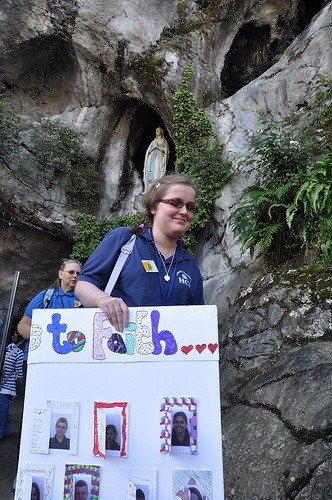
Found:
[159,199,199,212]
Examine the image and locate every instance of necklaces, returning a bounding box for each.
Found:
[157,244,178,283]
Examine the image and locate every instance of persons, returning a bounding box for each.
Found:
[136,489,145,500]
[140,127,170,195]
[0,324,25,442]
[73,174,206,332]
[18,257,85,382]
[170,410,190,447]
[74,480,90,500]
[31,482,40,500]
[188,486,203,500]
[48,416,70,450]
[105,424,121,451]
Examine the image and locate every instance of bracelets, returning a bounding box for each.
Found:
[95,295,114,307]
